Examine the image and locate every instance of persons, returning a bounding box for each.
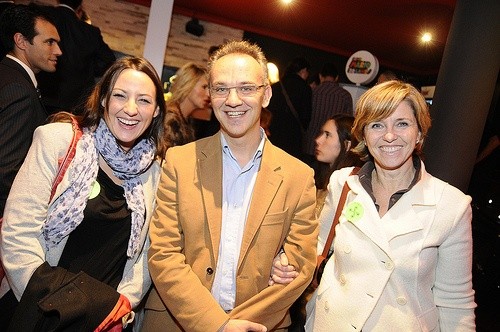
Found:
[0,0,500,222]
[268,80,477,332]
[0,56,166,332]
[140,42,317,332]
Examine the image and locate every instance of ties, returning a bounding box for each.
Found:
[36,87,41,100]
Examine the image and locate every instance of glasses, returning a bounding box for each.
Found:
[208,83,266,99]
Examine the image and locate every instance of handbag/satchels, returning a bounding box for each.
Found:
[297,256,326,326]
